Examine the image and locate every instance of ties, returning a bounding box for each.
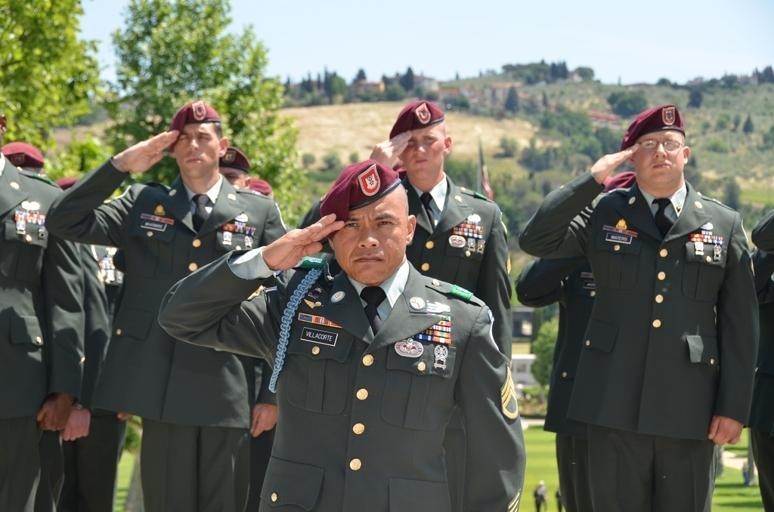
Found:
[651,199,674,237]
[192,194,211,233]
[419,191,435,231]
[360,287,386,336]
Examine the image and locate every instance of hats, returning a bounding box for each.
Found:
[600,170,636,191]
[321,160,402,237]
[218,146,251,174]
[55,175,75,188]
[620,105,686,150]
[245,179,273,197]
[389,102,444,140]
[393,160,408,180]
[168,103,222,153]
[4,142,44,170]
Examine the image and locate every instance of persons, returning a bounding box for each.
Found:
[300,101,511,366]
[0,101,286,512]
[520,105,760,510]
[516,171,637,511]
[158,159,527,511]
[748,210,773,512]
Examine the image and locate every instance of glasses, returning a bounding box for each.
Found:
[640,137,685,150]
